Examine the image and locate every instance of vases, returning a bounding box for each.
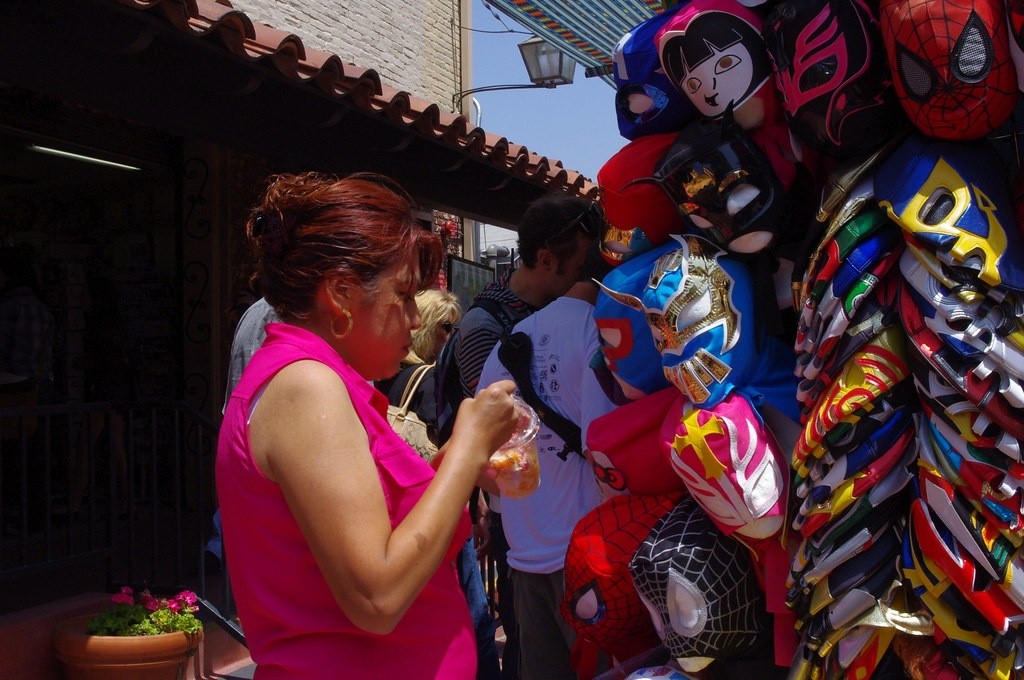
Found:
[53,614,204,680]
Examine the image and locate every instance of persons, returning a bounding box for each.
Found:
[214,170,519,680]
[455,195,635,680]
[221,297,286,416]
[371,286,502,680]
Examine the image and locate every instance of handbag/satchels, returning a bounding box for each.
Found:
[388,364,437,462]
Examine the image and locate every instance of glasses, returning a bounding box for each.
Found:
[545,201,606,250]
[438,322,459,334]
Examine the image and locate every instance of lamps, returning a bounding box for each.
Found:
[452,34,576,117]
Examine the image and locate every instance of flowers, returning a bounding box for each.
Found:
[88,583,204,637]
